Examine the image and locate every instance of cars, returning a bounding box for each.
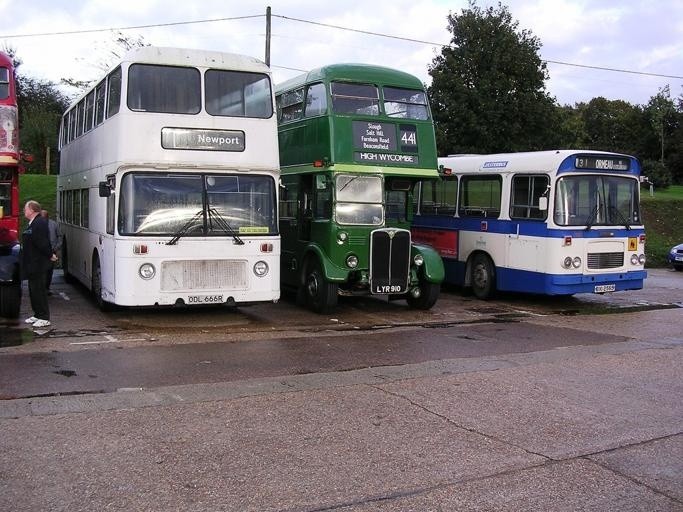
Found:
[667,242,683,272]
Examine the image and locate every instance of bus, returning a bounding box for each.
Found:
[0,50,33,323]
[410,147,657,303]
[55,45,285,316]
[275,62,451,315]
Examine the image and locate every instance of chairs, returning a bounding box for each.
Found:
[411,200,500,218]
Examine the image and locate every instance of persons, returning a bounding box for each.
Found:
[16,199,58,328]
[39,208,64,295]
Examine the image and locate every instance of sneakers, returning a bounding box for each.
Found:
[32,318,51,327]
[24,316,39,324]
